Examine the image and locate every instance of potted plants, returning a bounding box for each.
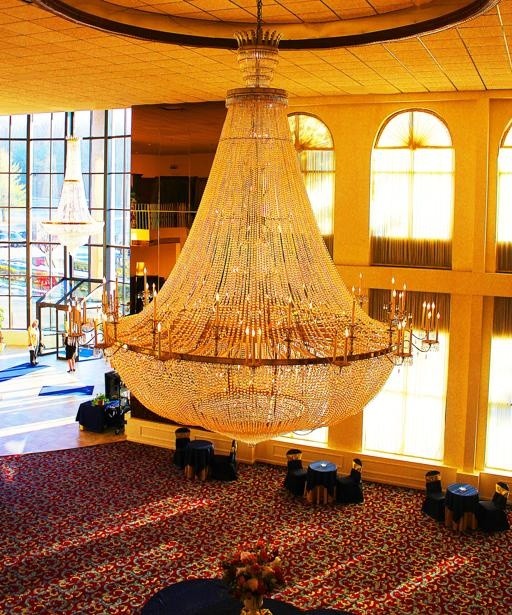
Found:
[91,393,109,407]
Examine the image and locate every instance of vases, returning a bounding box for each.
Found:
[243,597,262,610]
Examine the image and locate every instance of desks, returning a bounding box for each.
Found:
[142,577,304,613]
[79,396,128,434]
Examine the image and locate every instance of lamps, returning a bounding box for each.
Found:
[42,136,105,255]
[64,0,441,448]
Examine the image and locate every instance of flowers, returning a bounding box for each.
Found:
[212,538,294,601]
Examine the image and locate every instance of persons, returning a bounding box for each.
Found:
[28,319,39,366]
[63,328,76,373]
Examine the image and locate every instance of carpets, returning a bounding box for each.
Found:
[0,348,94,396]
[0,440,512,615]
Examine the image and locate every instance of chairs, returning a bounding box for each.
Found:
[284,449,363,505]
[422,470,510,532]
[172,428,237,482]
